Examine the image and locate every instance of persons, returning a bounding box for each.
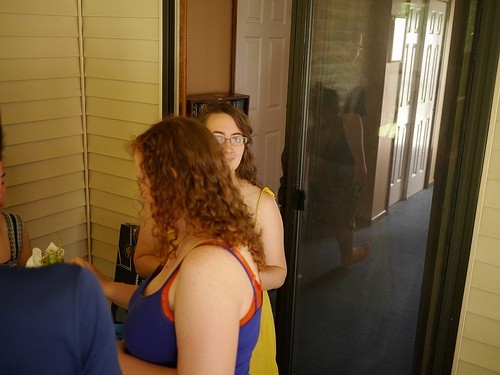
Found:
[302,18,374,266]
[0,261,124,375]
[72,116,280,375]
[132,100,288,292]
[0,110,34,268]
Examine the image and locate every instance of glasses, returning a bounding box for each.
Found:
[214,135,247,144]
